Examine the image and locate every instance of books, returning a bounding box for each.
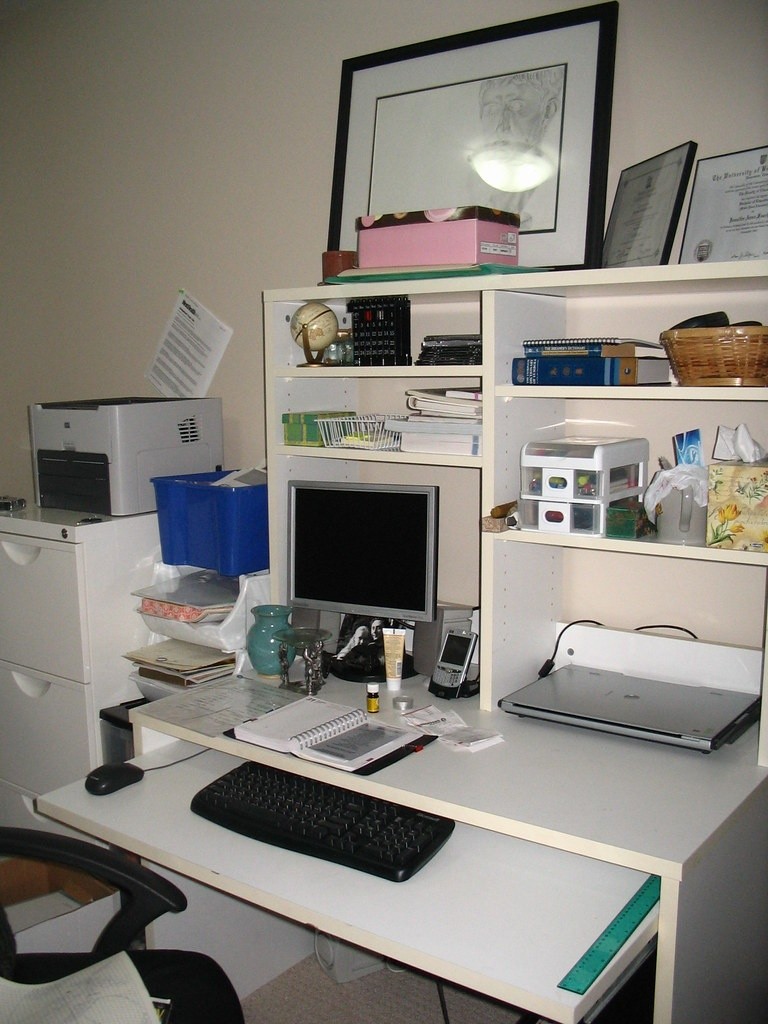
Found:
[384,388,483,456]
[512,337,672,386]
[130,570,240,623]
[223,695,439,775]
[122,639,236,687]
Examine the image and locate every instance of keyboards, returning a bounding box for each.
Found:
[190,761,456,882]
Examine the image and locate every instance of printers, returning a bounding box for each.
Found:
[29,397,224,517]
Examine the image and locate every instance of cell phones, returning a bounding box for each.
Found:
[433,627,479,688]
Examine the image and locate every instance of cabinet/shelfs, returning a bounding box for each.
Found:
[127,568,269,701]
[1,508,229,851]
[262,257,768,768]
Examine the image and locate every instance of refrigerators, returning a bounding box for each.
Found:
[0,503,209,859]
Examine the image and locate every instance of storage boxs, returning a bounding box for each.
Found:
[282,408,357,445]
[356,207,519,269]
[520,426,651,498]
[150,465,276,574]
[514,488,643,537]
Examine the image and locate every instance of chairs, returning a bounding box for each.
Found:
[0,813,244,1024]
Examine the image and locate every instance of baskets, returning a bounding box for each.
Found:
[658,327,768,387]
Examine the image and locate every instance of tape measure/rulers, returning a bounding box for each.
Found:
[558,874,661,996]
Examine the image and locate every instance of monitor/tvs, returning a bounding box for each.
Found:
[286,479,439,684]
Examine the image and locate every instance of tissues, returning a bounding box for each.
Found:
[705,423,768,553]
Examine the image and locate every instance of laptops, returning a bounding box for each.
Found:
[498,664,760,753]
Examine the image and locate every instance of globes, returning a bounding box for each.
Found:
[290,303,339,368]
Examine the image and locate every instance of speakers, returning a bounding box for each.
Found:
[413,604,473,677]
[315,929,386,984]
[291,607,341,653]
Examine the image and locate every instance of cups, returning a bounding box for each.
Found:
[322,251,357,284]
[656,481,709,547]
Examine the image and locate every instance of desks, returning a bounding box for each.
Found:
[34,662,768,1024]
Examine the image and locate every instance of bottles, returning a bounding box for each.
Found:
[366,682,380,713]
[246,605,294,679]
[324,328,355,366]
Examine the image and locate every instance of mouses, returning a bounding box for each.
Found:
[85,763,144,796]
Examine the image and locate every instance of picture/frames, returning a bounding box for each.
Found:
[327,2,621,276]
[599,141,697,268]
[679,146,767,265]
